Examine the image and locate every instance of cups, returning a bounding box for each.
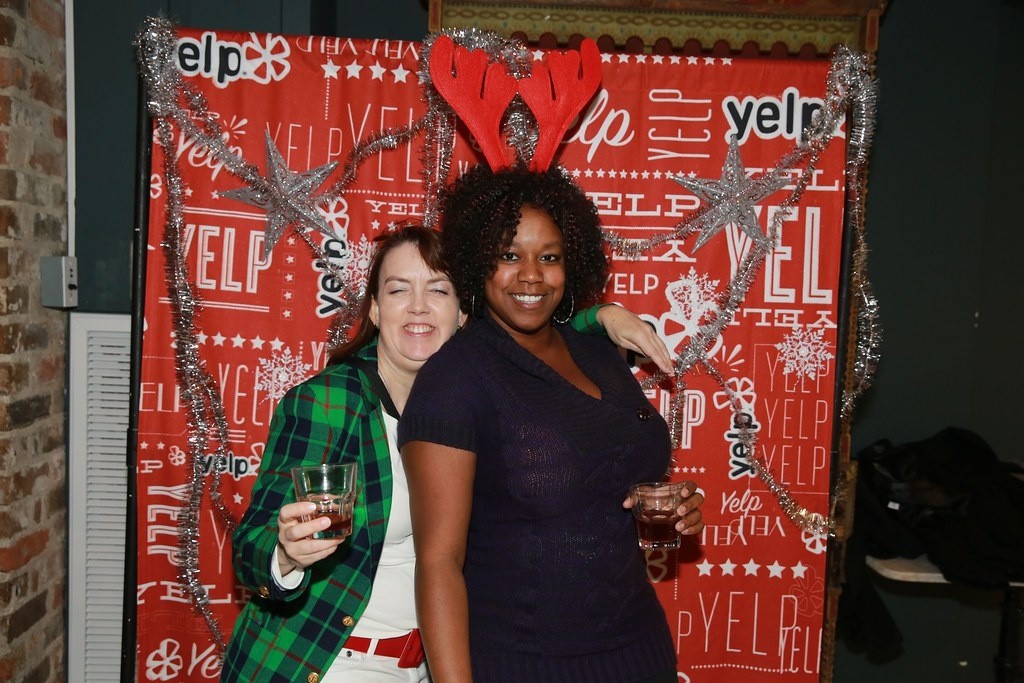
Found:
[290,462,358,541]
[629,481,684,550]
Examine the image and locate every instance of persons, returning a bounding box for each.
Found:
[215,225,676,682]
[399,164,705,682]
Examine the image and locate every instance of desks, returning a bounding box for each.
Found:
[866,553,1024,683]
